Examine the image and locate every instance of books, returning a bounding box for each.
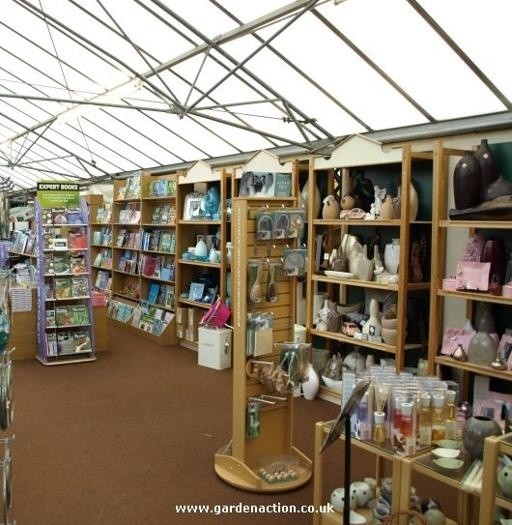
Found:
[44,176,178,357]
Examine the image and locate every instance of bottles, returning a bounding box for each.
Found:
[301,362,320,401]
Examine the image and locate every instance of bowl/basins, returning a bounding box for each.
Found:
[428,439,466,474]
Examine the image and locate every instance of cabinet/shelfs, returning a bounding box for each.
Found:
[6,133,512,525]
